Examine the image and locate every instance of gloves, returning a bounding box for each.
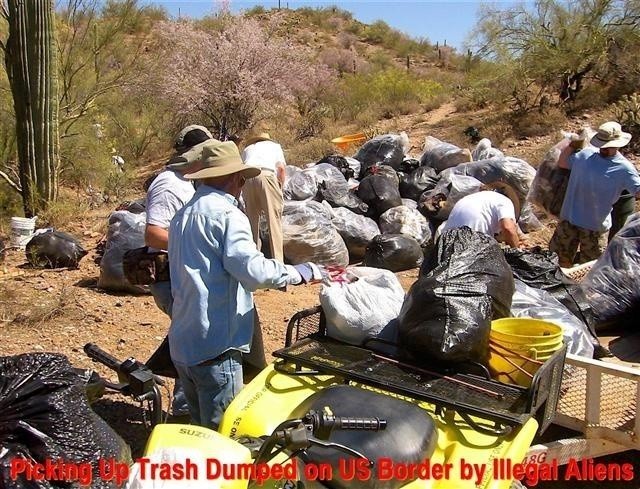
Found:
[293,261,334,288]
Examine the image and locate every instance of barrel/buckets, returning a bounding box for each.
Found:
[10,216,36,251]
[221,352,541,489]
[487,317,564,391]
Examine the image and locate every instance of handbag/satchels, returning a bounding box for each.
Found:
[123,246,169,285]
[145,333,179,378]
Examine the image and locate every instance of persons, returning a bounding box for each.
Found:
[168,140,344,431]
[549,121,640,268]
[441,181,520,249]
[241,133,286,291]
[145,124,222,416]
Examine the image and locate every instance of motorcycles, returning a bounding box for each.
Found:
[83,298,640,489]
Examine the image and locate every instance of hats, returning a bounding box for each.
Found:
[590,120,632,148]
[479,181,520,223]
[109,147,119,154]
[165,125,221,171]
[183,141,261,179]
[245,132,278,146]
